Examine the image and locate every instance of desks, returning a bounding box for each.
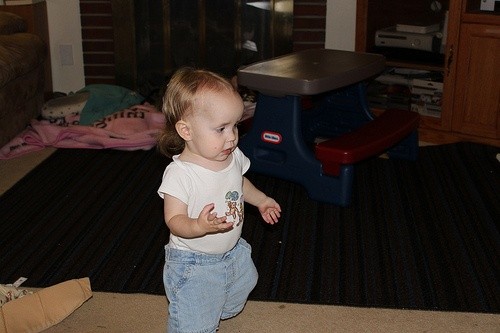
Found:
[238,48,385,207]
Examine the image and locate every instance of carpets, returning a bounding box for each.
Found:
[1,141,500,313]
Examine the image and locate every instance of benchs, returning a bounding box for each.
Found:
[317,109,422,177]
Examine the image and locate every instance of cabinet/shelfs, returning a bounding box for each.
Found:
[355,0,500,146]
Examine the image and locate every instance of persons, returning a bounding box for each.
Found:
[158,66,281,333]
